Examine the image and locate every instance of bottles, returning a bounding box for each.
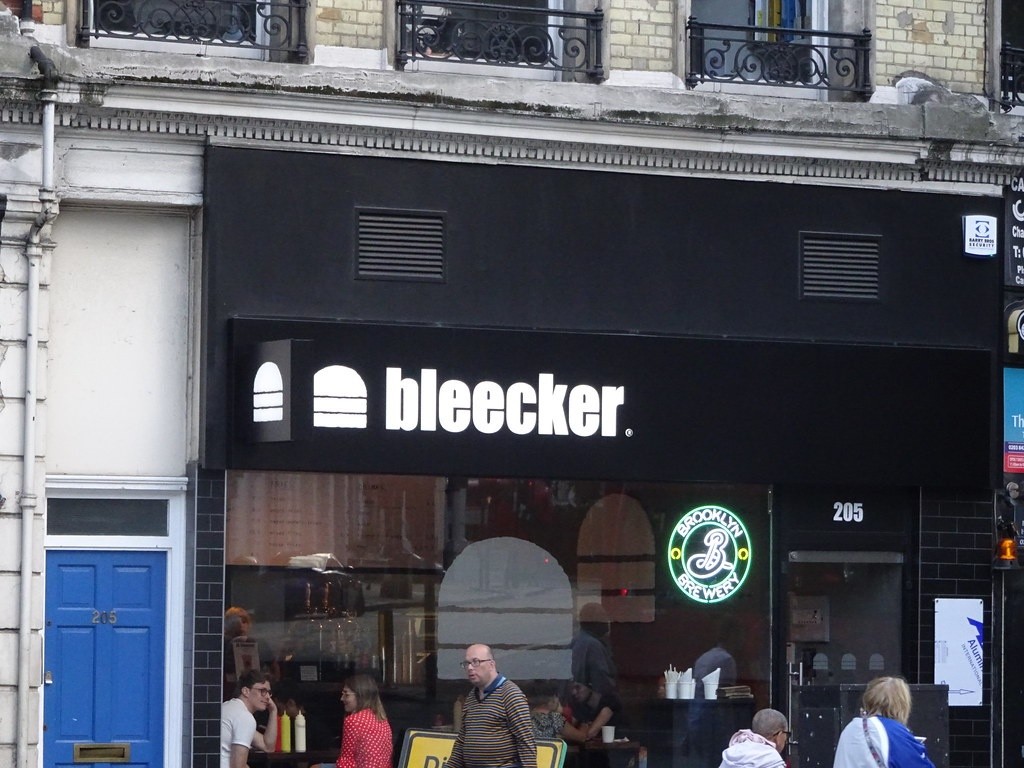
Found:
[276,716,281,752]
[295,709,306,753]
[280,711,292,753]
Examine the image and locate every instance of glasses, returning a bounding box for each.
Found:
[773,731,792,739]
[248,687,272,698]
[460,659,492,669]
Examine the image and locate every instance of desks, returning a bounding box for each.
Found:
[249,748,342,768]
[566,738,639,768]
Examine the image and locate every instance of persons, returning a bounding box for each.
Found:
[219,668,278,768]
[686,611,750,768]
[718,707,790,768]
[570,668,628,739]
[309,673,394,768]
[556,601,626,718]
[444,644,538,768]
[223,607,275,673]
[832,677,937,768]
[526,674,590,743]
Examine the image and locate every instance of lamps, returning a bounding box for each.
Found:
[992,519,1021,569]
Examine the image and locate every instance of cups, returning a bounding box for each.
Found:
[704,683,719,700]
[677,682,691,700]
[601,726,615,743]
[690,682,697,699]
[664,682,678,700]
